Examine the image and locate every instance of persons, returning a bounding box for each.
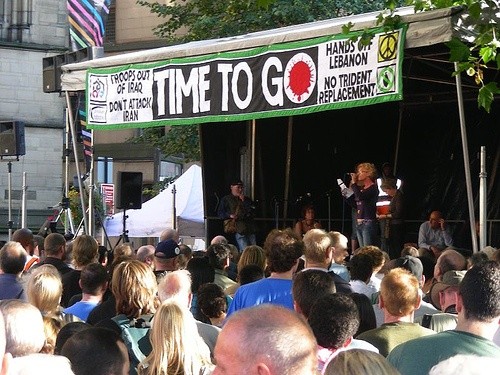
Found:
[418,210,453,257]
[0,228,500,375]
[341,162,407,261]
[218,179,257,253]
[451,207,488,254]
[295,206,321,242]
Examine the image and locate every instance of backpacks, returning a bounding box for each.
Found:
[112,312,154,375]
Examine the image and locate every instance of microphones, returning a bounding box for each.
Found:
[346,173,358,179]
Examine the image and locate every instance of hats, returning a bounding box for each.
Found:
[229,178,243,185]
[429,270,467,310]
[153,239,182,258]
[386,255,424,280]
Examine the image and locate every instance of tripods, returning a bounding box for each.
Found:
[110,209,129,254]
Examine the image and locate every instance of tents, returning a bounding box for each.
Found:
[103,164,204,237]
[60,0,500,253]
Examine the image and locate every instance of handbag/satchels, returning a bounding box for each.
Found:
[223,218,237,234]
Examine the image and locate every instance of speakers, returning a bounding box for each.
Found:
[0,120,26,157]
[116,170,143,210]
[42,55,65,93]
